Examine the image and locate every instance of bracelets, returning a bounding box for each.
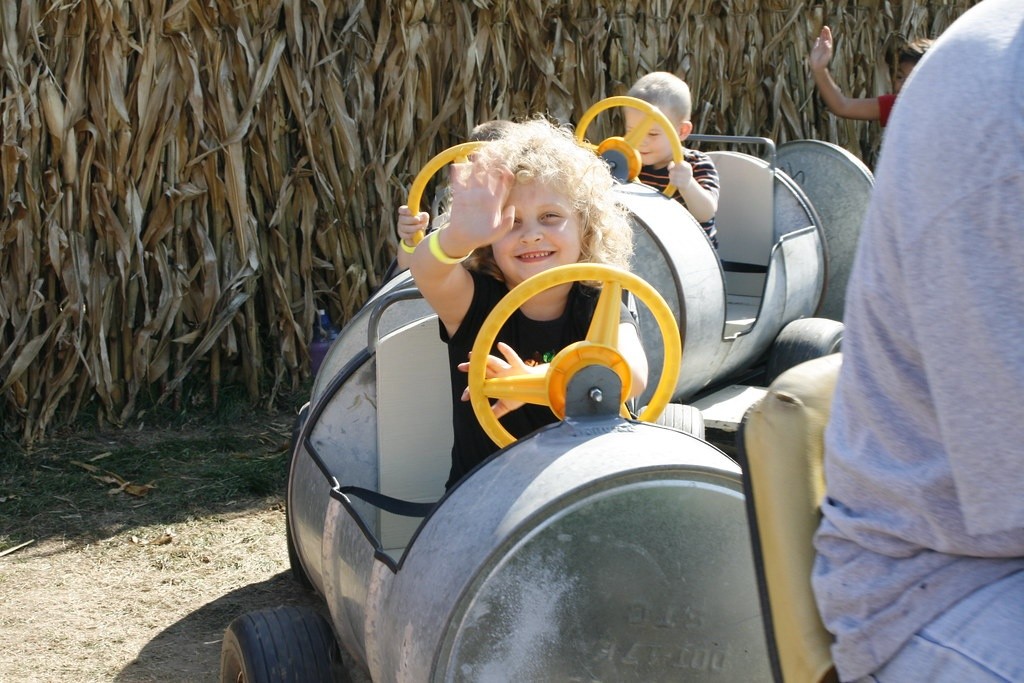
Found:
[430,228,473,265]
[400,239,415,254]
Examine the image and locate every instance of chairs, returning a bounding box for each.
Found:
[364,133,779,551]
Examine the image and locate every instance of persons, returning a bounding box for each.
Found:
[622,72,719,249]
[410,120,647,493]
[397,120,520,271]
[809,25,935,127]
[811,0,1024,683]
